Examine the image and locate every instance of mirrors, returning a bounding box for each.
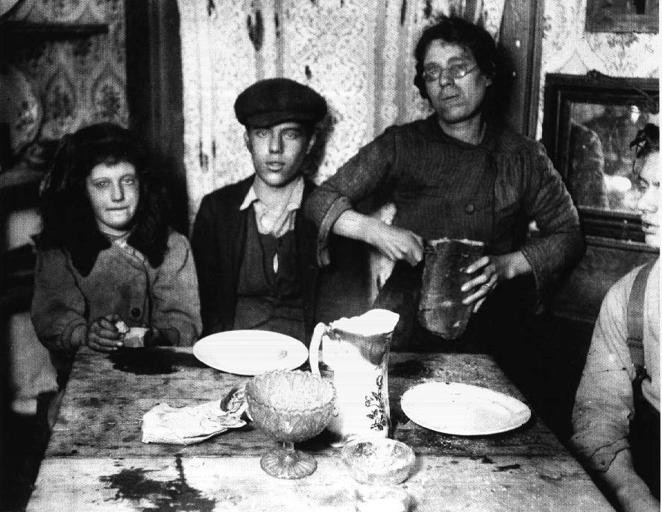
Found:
[534,72,660,236]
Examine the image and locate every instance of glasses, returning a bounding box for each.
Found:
[423,61,479,81]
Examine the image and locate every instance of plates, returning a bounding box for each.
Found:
[399,378,532,440]
[192,329,309,379]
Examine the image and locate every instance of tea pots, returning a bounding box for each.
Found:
[307,309,401,443]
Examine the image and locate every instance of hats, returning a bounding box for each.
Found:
[234,78,327,128]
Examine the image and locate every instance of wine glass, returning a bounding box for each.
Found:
[245,370,336,482]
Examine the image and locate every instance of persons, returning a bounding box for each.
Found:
[568,118,612,211]
[302,10,590,359]
[191,74,372,374]
[617,102,643,163]
[30,118,203,394]
[565,118,660,512]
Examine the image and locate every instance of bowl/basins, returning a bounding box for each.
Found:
[341,435,416,486]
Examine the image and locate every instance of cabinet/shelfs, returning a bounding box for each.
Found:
[1,0,180,325]
